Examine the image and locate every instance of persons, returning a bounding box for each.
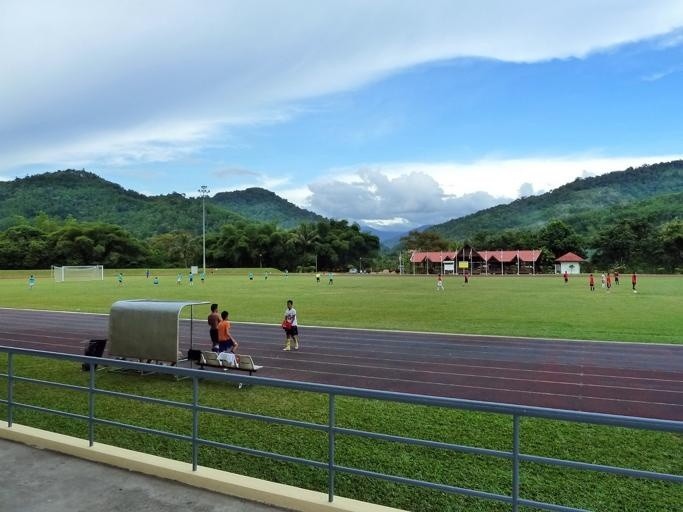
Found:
[316,272,320,283]
[200,272,205,284]
[285,271,288,281]
[218,311,240,354]
[28,275,36,290]
[118,273,124,287]
[264,272,267,280]
[463,273,469,287]
[328,272,334,285]
[282,300,299,351]
[188,272,193,286]
[153,277,159,286]
[146,270,150,280]
[249,272,254,280]
[436,274,444,291]
[588,271,619,291]
[176,272,183,288]
[563,271,569,286]
[631,271,637,290]
[208,303,222,352]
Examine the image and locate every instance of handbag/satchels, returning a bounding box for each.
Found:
[282,320,292,329]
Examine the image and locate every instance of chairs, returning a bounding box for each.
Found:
[189,349,263,376]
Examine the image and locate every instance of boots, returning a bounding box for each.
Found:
[294,338,298,350]
[282,340,291,351]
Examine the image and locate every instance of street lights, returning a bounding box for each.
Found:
[198,184,210,274]
